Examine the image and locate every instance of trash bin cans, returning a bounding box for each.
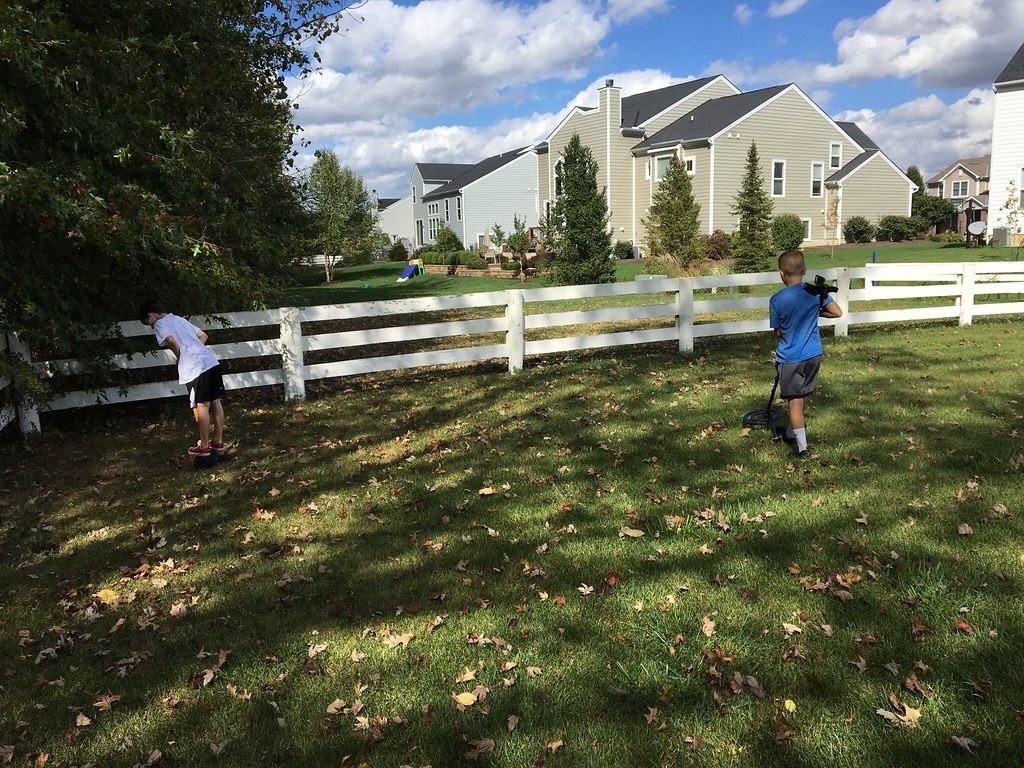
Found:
[993,226,1010,248]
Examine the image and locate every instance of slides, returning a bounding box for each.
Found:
[395,264,418,283]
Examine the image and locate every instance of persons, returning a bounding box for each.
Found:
[768,250,843,456]
[140,301,225,456]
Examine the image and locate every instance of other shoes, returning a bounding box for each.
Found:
[774,426,797,446]
[789,449,810,458]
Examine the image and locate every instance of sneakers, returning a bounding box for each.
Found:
[188,443,211,456]
[197,439,224,450]
